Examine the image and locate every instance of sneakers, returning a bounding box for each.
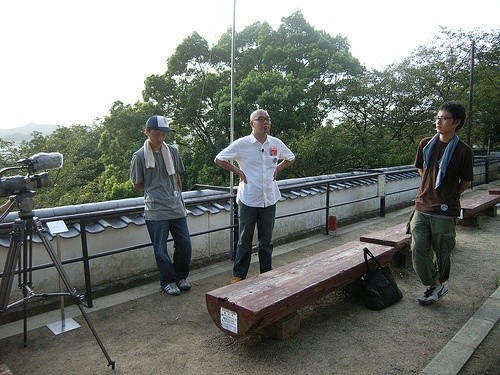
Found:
[163,282,180,294]
[418,284,449,305]
[176,279,191,289]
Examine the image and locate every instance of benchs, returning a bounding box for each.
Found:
[360,221,413,269]
[205,240,395,340]
[457,186,500,227]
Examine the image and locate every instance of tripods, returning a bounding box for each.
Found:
[0,190,115,370]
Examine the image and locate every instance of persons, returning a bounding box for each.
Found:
[129,115,192,296]
[213,108,295,287]
[411,102,475,305]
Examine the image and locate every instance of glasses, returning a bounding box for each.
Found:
[434,116,454,122]
[253,118,271,122]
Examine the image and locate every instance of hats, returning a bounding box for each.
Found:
[146,116,176,133]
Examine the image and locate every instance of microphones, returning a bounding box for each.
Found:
[27,151,63,170]
[261,148,264,152]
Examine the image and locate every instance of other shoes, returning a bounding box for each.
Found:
[230,277,241,283]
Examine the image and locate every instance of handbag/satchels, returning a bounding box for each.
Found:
[356,248,403,311]
